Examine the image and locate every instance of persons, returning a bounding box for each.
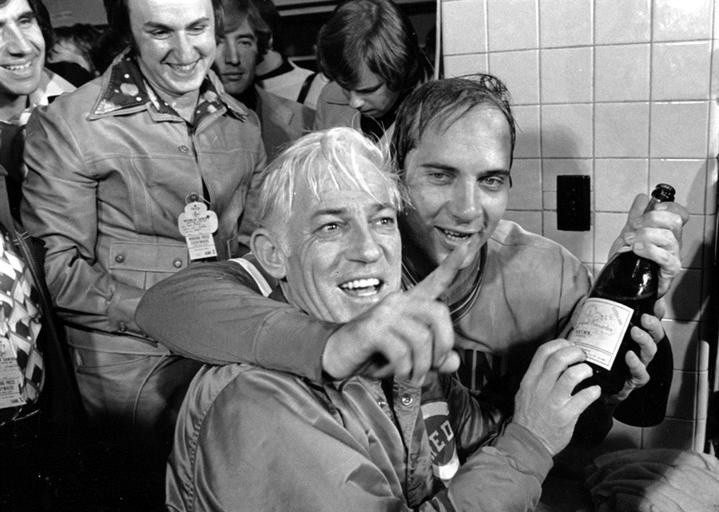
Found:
[0,1,436,427]
[132,70,691,511]
[163,122,666,511]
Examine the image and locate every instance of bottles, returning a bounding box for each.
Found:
[566,183,676,389]
